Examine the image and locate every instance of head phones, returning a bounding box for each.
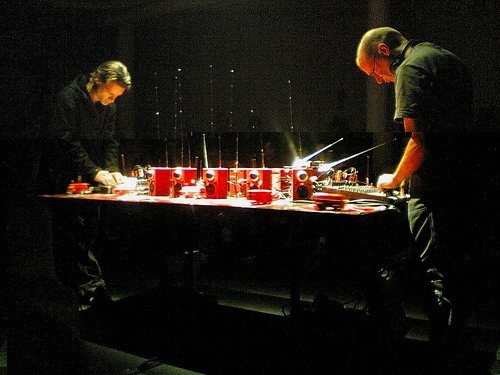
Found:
[390,38,415,75]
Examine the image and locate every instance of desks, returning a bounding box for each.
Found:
[37,192,407,375]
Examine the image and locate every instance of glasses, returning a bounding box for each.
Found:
[369,48,379,80]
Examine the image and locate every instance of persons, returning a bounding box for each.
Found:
[354,27,500,375]
[34,60,133,314]
